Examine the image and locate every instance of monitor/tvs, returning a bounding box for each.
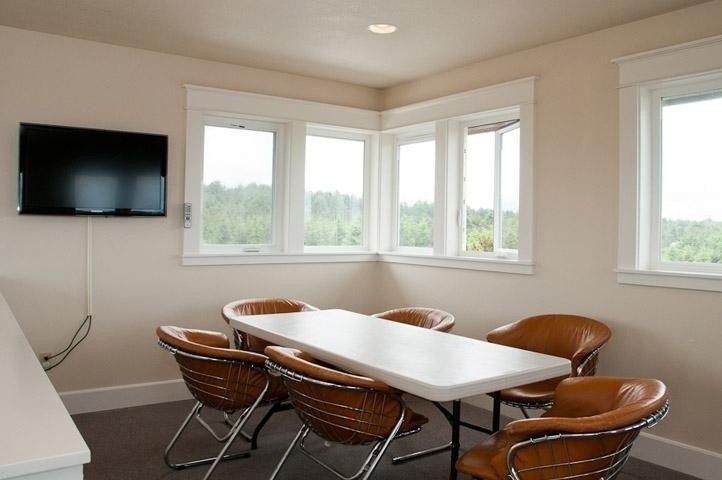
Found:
[17,123,168,216]
